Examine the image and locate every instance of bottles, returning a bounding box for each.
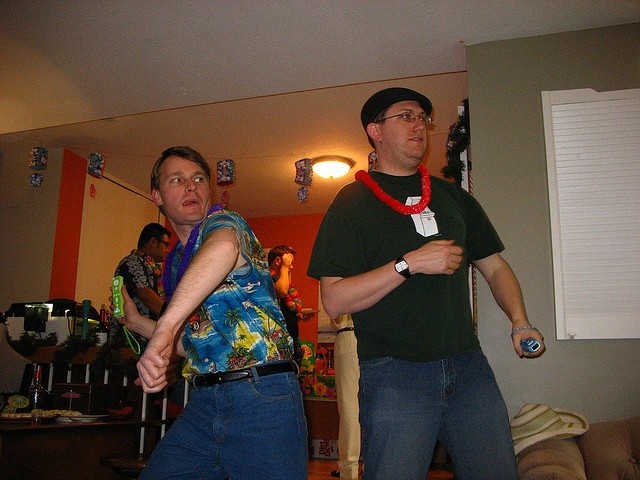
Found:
[28,365,44,428]
[94,304,108,347]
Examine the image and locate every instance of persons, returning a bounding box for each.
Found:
[108,144,311,480]
[108,221,174,344]
[262,245,315,365]
[312,86,547,479]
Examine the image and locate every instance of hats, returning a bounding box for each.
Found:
[510,403,589,455]
[361,87,433,129]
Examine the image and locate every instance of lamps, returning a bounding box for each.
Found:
[308,154,355,182]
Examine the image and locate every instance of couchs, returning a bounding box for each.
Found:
[516,416,639,479]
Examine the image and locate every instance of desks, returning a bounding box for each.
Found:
[0,418,168,479]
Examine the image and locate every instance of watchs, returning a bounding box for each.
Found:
[391,256,412,280]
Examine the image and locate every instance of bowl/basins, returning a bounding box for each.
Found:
[57,416,73,423]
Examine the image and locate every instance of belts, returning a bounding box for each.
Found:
[194,361,296,387]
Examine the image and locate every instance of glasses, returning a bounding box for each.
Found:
[376,112,433,125]
[157,238,169,246]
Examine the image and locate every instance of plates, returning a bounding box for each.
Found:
[61,415,110,422]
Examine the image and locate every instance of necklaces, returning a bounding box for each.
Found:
[162,205,223,301]
[355,162,432,215]
